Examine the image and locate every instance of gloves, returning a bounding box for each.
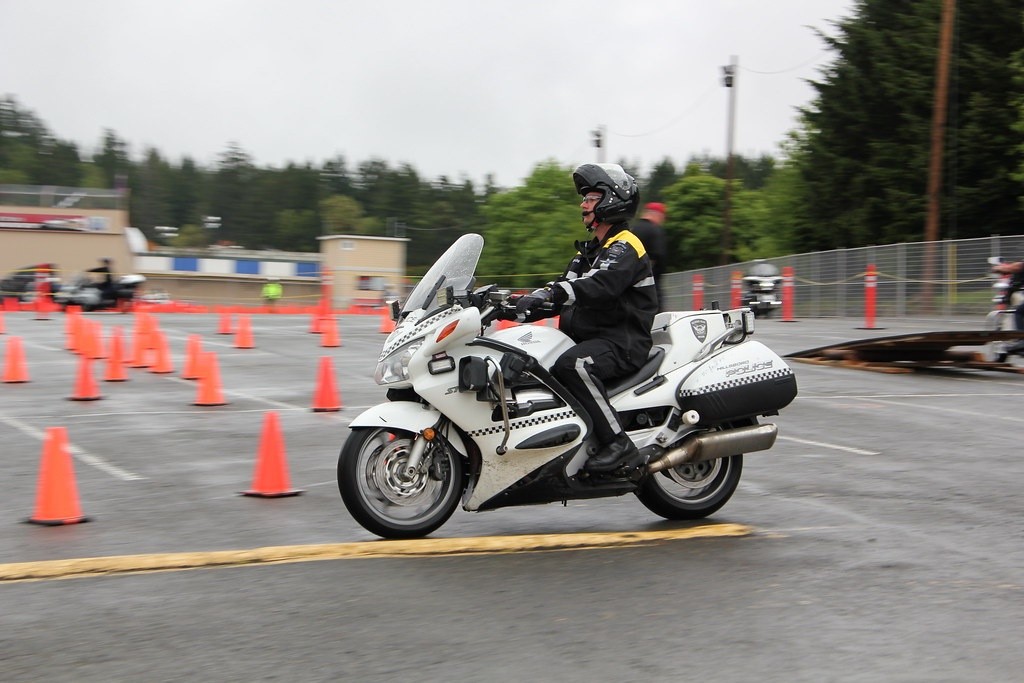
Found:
[516,284,568,323]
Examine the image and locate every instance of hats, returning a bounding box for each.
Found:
[647,202,665,213]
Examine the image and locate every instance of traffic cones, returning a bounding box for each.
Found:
[0,335,33,384]
[189,352,230,408]
[64,353,106,402]
[380,305,397,335]
[310,294,342,347]
[21,428,96,529]
[311,355,344,411]
[241,410,306,502]
[215,305,257,349]
[0,295,202,381]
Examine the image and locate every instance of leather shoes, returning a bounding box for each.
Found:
[585,439,639,471]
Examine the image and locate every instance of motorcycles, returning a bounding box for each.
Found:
[337,234,797,539]
[52,271,148,311]
[983,256,1024,363]
[741,277,782,318]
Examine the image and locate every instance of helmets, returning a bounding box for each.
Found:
[573,163,639,222]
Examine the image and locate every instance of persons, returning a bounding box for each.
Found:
[497,162,658,473]
[85,258,113,299]
[990,260,1024,354]
[633,202,667,292]
[262,278,282,312]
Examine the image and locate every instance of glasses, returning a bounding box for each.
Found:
[582,195,601,204]
